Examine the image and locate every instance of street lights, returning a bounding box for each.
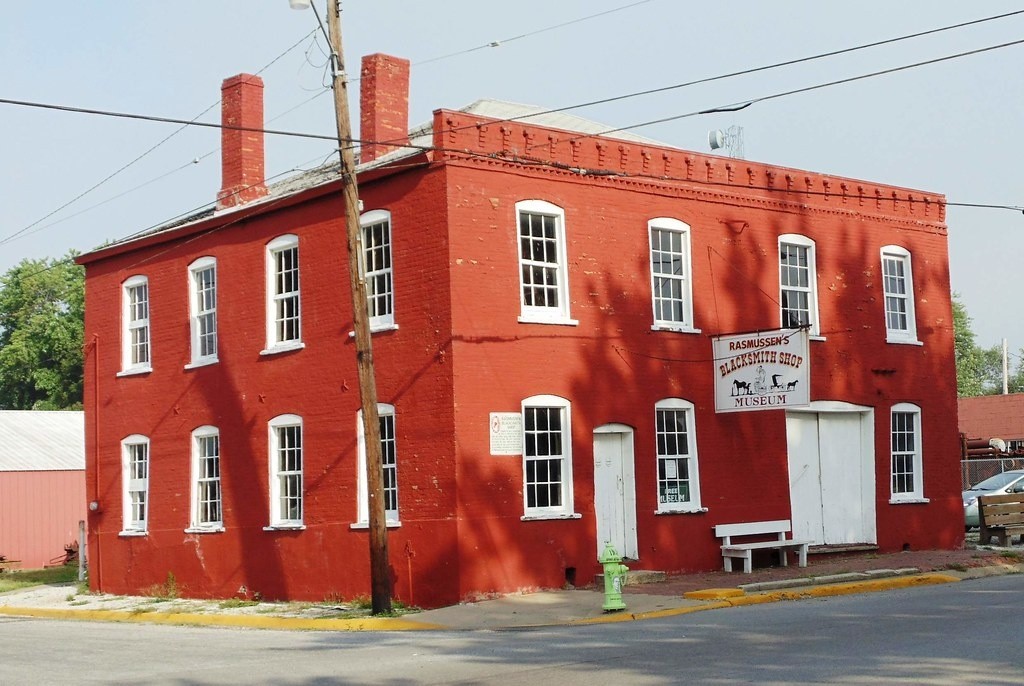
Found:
[287,1,400,615]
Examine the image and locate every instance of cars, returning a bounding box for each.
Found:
[961,469,1024,533]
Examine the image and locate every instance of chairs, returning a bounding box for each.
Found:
[711,519,812,574]
[977,492,1024,549]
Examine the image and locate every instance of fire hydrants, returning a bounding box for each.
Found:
[599,544,630,612]
